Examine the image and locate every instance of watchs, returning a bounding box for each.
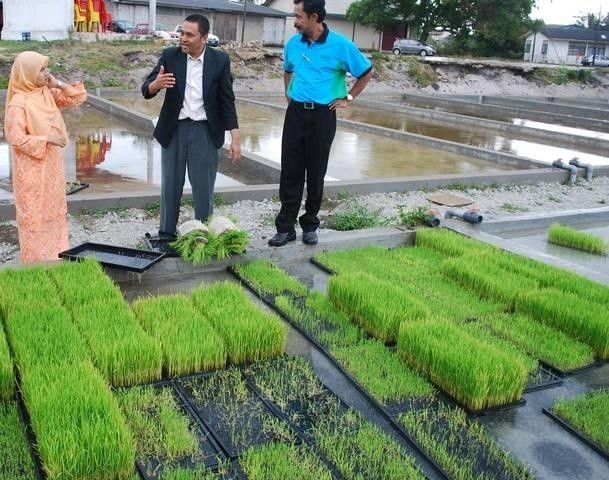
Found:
[346,93,353,101]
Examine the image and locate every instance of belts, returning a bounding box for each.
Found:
[291,98,330,110]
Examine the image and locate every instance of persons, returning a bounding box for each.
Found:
[140,14,242,242]
[3,50,88,265]
[268,0,374,249]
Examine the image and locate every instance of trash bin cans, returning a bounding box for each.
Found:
[22,32,31,41]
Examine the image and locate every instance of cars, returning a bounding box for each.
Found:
[107,20,219,47]
[392,37,438,56]
[581,55,608,66]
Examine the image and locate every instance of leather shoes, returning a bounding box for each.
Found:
[268,229,296,246]
[303,230,318,245]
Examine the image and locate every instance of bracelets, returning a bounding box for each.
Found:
[56,79,62,88]
